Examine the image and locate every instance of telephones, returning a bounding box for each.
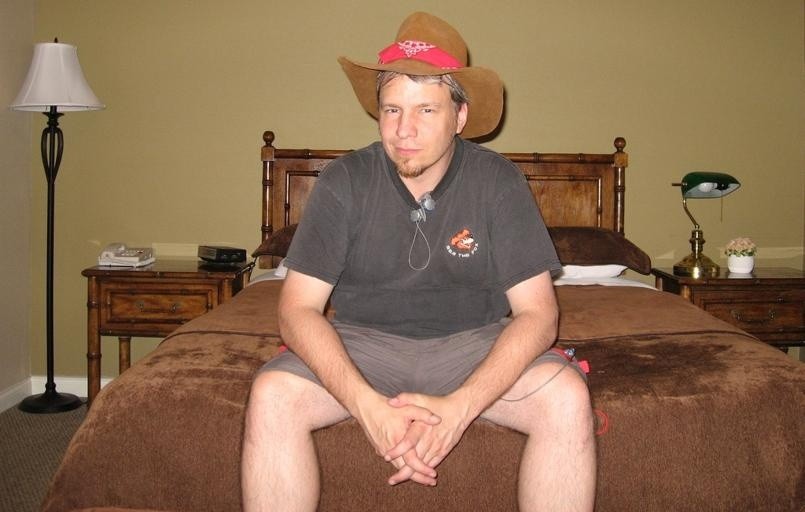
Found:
[98,243,156,266]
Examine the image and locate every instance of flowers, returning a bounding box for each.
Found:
[725,236,759,257]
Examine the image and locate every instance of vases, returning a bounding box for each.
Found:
[728,273,752,278]
[727,254,754,273]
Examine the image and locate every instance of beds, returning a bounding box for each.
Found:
[99,130,801,511]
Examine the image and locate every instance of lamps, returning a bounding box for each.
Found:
[671,171,741,274]
[7,36,106,414]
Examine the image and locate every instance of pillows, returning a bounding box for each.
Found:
[251,223,299,259]
[546,225,651,279]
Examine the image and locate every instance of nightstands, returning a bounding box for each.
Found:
[81,258,256,415]
[650,266,804,355]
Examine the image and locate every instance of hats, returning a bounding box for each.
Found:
[337,11,504,139]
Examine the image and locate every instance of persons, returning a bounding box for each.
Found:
[238,9,599,512]
